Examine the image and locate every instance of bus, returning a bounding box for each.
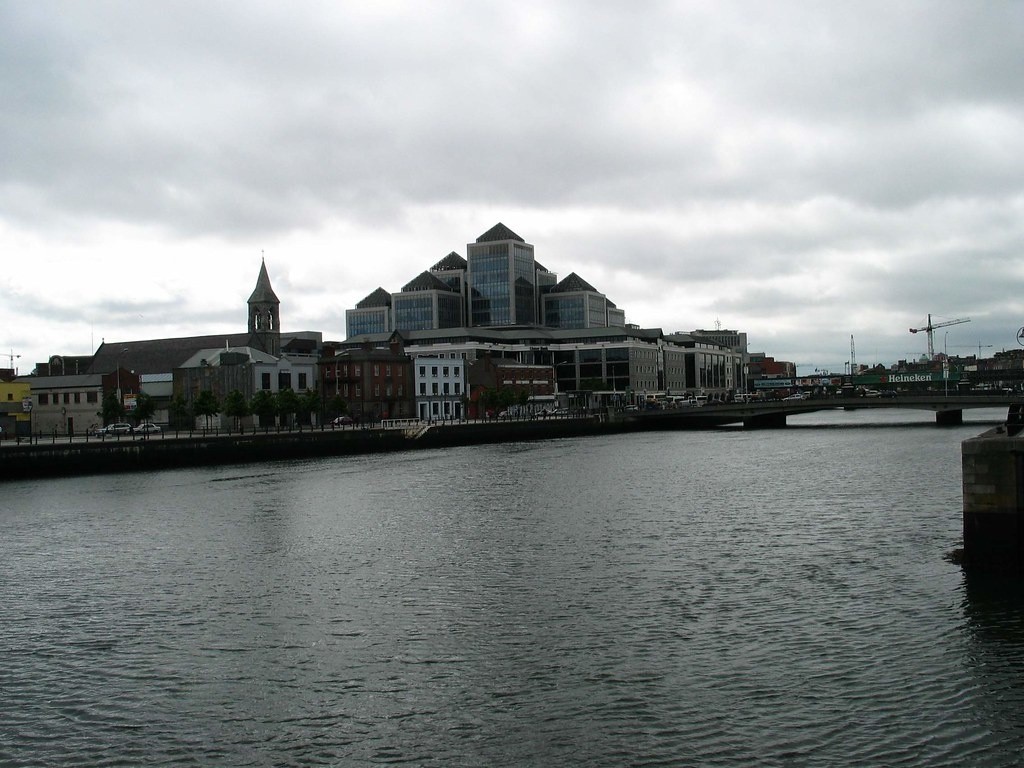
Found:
[667,396,683,406]
[646,393,667,408]
[688,396,708,403]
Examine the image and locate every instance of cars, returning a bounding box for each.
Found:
[95,423,133,436]
[535,409,551,417]
[330,417,355,427]
[784,393,805,402]
[867,391,879,398]
[877,391,898,399]
[133,423,162,434]
[618,405,640,413]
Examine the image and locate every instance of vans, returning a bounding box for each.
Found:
[550,408,569,416]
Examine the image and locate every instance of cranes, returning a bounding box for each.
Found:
[850,334,855,364]
[909,314,974,361]
[0,350,21,369]
[950,341,993,359]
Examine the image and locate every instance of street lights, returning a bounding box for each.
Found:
[552,360,568,387]
[944,331,949,397]
[115,348,129,422]
